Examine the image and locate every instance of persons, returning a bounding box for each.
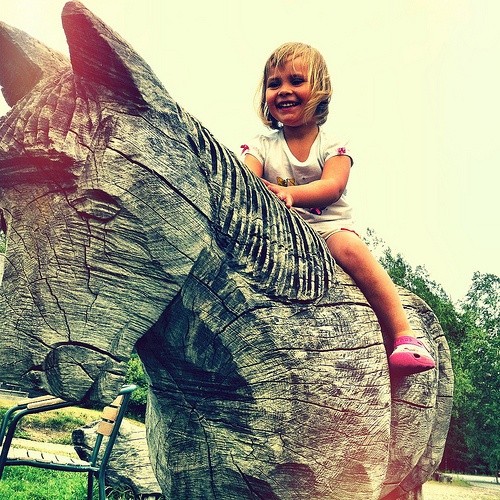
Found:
[240,42,435,375]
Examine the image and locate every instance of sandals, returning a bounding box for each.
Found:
[388,337,435,371]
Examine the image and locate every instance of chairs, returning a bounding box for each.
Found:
[0,384,137,500]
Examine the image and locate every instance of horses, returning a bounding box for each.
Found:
[0,0,454,500]
